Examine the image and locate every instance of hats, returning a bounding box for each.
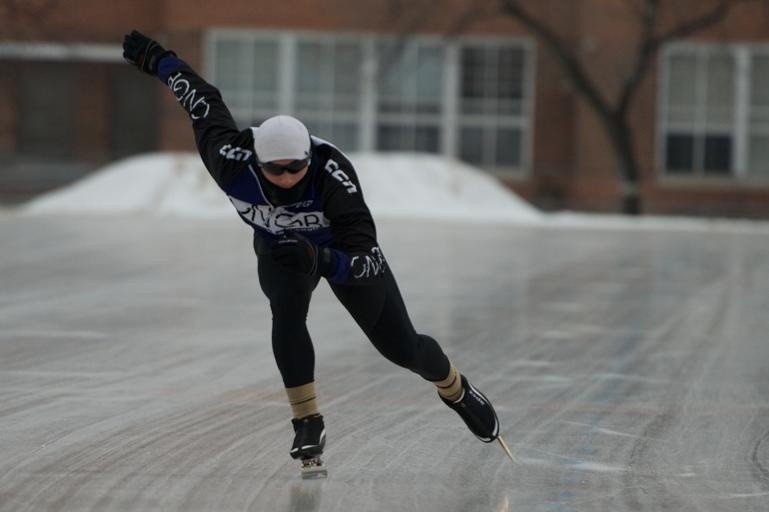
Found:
[253,114,312,164]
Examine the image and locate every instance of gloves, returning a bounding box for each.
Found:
[265,230,333,277]
[122,28,178,80]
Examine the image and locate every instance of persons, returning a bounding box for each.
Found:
[121,28,501,462]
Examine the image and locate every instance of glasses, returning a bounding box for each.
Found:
[258,156,311,176]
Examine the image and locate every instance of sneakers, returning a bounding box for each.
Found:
[289,412,327,460]
[437,371,500,444]
